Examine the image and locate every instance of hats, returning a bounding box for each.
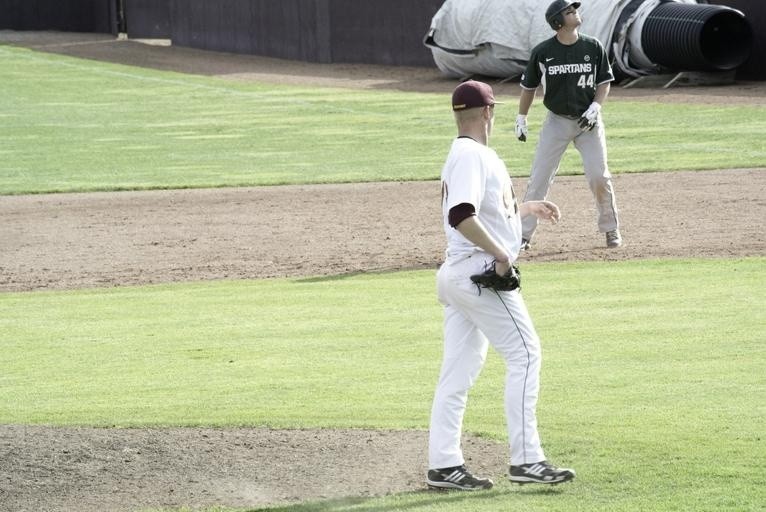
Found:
[453,79,504,110]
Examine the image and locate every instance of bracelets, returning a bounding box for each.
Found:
[494,259,509,266]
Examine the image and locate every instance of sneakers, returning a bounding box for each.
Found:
[520,238,531,250]
[427,464,493,491]
[510,461,574,483]
[606,229,622,248]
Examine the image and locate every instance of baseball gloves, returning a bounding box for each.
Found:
[470,260,520,296]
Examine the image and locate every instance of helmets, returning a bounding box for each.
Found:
[546,0,581,29]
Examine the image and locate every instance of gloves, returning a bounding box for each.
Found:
[516,113,529,142]
[578,101,602,132]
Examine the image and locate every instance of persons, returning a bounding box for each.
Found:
[515,1,622,252]
[427,79,574,492]
